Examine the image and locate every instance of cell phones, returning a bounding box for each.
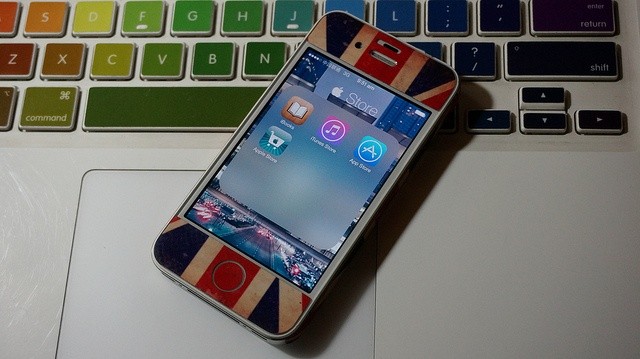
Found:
[150,9,462,343]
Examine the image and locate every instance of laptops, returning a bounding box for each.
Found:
[0,1,639,358]
